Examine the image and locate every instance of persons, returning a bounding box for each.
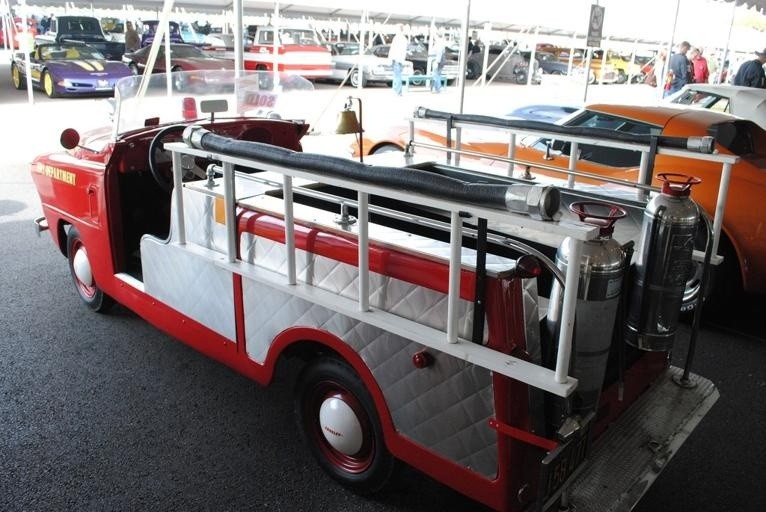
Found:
[47,13,53,30]
[469,31,481,78]
[193,21,199,32]
[204,21,211,35]
[41,16,47,33]
[654,39,766,99]
[428,35,445,93]
[387,24,407,96]
[124,21,140,53]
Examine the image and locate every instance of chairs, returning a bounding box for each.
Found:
[66,49,80,59]
[34,47,51,60]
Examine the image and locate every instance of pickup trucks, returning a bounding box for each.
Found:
[35,16,126,61]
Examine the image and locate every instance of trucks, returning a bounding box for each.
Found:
[29,70,717,507]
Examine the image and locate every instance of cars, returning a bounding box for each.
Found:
[466,45,656,86]
[11,44,137,98]
